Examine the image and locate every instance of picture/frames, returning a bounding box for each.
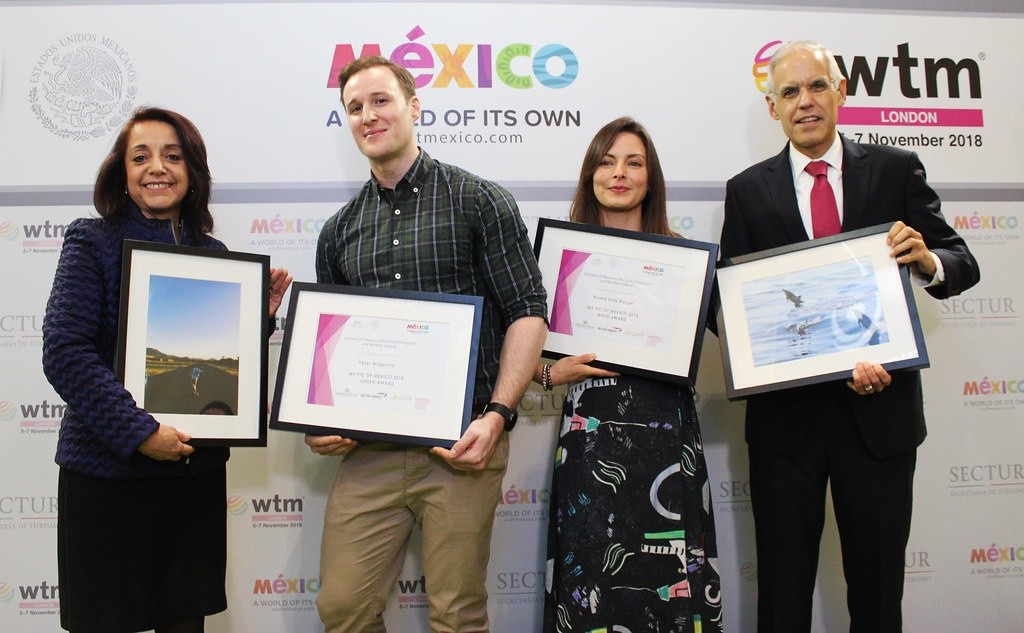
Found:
[112,238,268,450]
[269,282,486,451]
[529,216,720,387]
[715,221,930,402]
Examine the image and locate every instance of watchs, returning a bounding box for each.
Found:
[477,402,518,431]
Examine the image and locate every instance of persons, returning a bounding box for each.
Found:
[42,107,293,633]
[720,40,981,633]
[304,55,549,633]
[532,116,719,633]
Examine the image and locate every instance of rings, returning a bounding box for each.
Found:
[864,384,873,391]
[270,286,279,295]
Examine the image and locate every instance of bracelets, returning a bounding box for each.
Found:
[542,364,553,391]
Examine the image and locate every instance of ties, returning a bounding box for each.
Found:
[805,160,841,240]
